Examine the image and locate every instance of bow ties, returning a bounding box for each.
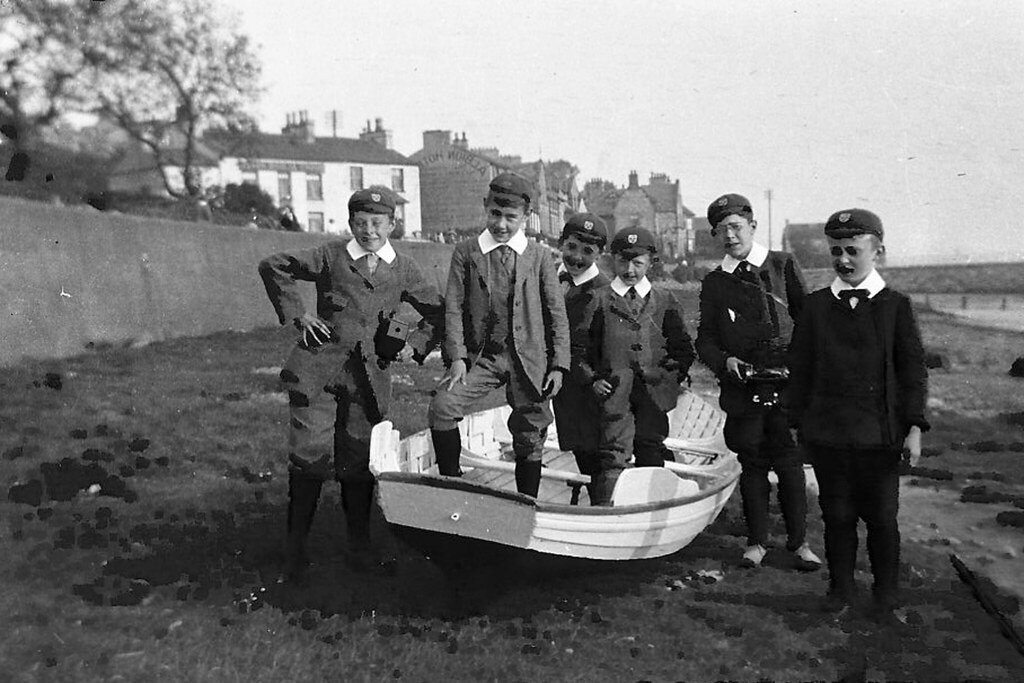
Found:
[557,270,576,287]
[838,288,869,304]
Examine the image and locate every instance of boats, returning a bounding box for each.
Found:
[368,377,743,563]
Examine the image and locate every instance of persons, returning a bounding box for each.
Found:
[784,210,930,613]
[259,186,444,582]
[431,173,573,501]
[695,194,822,569]
[540,213,695,507]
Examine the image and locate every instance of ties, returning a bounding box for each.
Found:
[739,260,748,273]
[498,244,510,264]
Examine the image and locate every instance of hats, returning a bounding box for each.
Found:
[610,225,658,257]
[348,186,396,218]
[823,208,884,241]
[489,173,534,207]
[707,194,754,237]
[562,212,609,251]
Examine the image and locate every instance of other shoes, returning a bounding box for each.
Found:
[786,541,823,571]
[873,592,906,624]
[741,539,772,567]
[820,593,846,612]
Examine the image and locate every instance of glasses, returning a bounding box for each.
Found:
[713,221,744,236]
